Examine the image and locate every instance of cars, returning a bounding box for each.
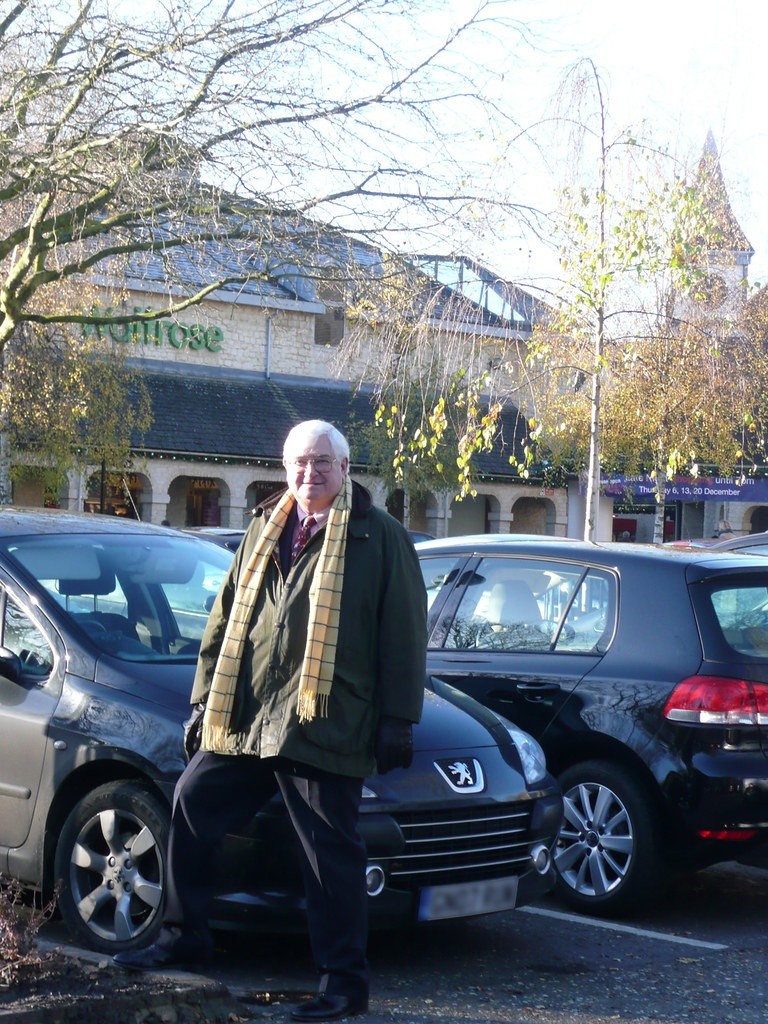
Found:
[1,501,568,970]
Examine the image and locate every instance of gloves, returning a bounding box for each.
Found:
[184,702,208,764]
[367,716,413,776]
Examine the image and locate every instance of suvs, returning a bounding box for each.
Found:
[173,513,768,918]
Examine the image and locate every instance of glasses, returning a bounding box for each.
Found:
[287,457,338,473]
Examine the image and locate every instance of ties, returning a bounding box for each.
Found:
[290,514,318,564]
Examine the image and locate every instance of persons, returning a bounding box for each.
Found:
[159,519,172,526]
[715,519,736,542]
[112,414,426,1024]
[616,530,633,543]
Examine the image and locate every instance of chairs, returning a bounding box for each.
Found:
[470,581,557,656]
[58,560,145,665]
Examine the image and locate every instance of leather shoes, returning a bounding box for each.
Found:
[113,931,214,972]
[288,987,371,1024]
